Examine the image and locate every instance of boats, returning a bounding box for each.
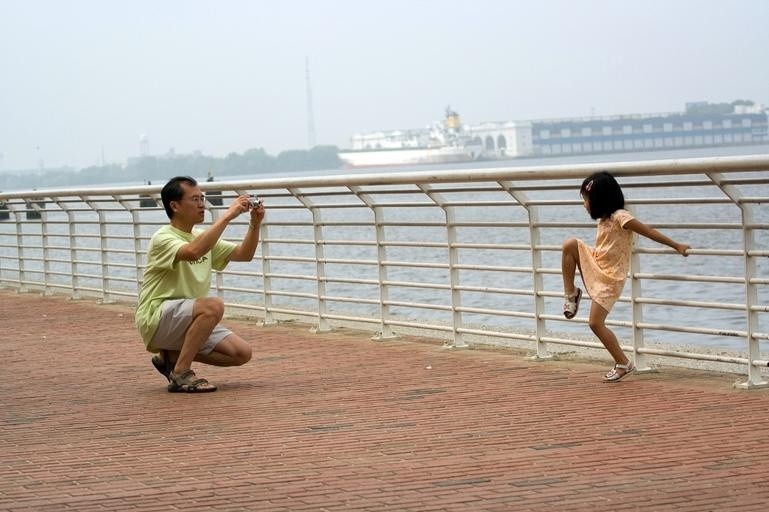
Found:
[206,178,223,205]
[26,194,45,219]
[140,181,156,206]
[1,198,9,219]
[338,111,484,165]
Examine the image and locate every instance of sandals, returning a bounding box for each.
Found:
[603,360,637,382]
[563,287,582,319]
[152,350,217,392]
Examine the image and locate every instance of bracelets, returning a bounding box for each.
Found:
[249,221,260,230]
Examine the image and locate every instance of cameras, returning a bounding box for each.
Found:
[248,196,263,209]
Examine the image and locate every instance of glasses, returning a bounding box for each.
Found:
[179,195,206,204]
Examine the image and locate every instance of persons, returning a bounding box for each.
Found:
[134,176,264,394]
[559,172,692,381]
